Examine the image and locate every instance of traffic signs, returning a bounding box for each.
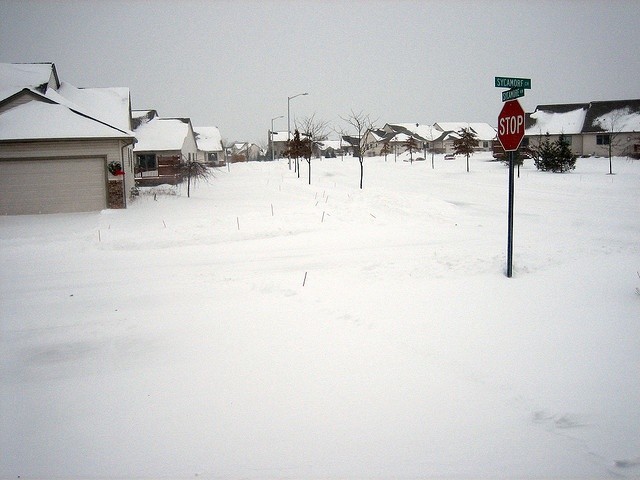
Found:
[502,89,524,102]
[495,77,531,89]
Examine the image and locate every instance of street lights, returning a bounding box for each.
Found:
[288,93,308,170]
[271,116,284,161]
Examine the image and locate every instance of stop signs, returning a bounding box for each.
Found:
[497,100,524,151]
